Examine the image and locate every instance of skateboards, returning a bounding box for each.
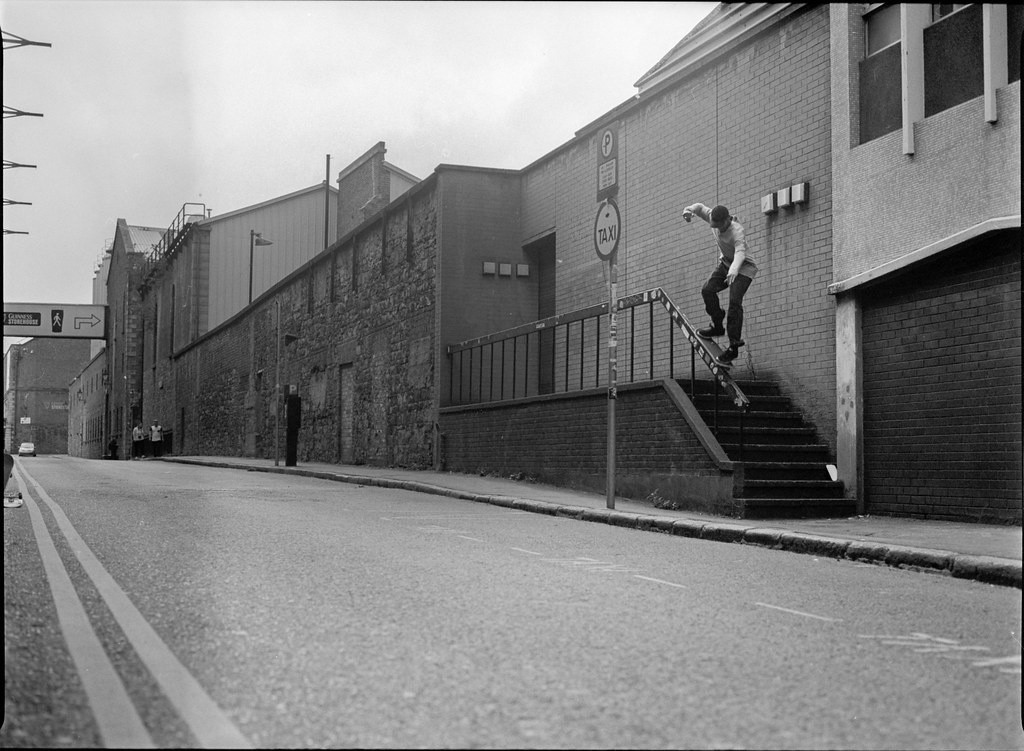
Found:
[694,327,734,371]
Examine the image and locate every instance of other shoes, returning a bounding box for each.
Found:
[135,456,138,460]
[142,455,146,458]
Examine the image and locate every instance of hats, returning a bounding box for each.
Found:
[710,205,728,228]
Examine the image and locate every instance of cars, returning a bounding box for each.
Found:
[17,442,36,457]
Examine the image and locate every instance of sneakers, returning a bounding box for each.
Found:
[697,322,726,338]
[716,347,738,364]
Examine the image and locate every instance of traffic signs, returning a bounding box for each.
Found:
[3,302,106,339]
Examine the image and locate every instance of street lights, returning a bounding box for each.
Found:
[249,230,273,304]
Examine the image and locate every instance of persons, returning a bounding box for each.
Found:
[108,438,117,459]
[149,420,164,458]
[681,203,759,364]
[133,422,146,460]
